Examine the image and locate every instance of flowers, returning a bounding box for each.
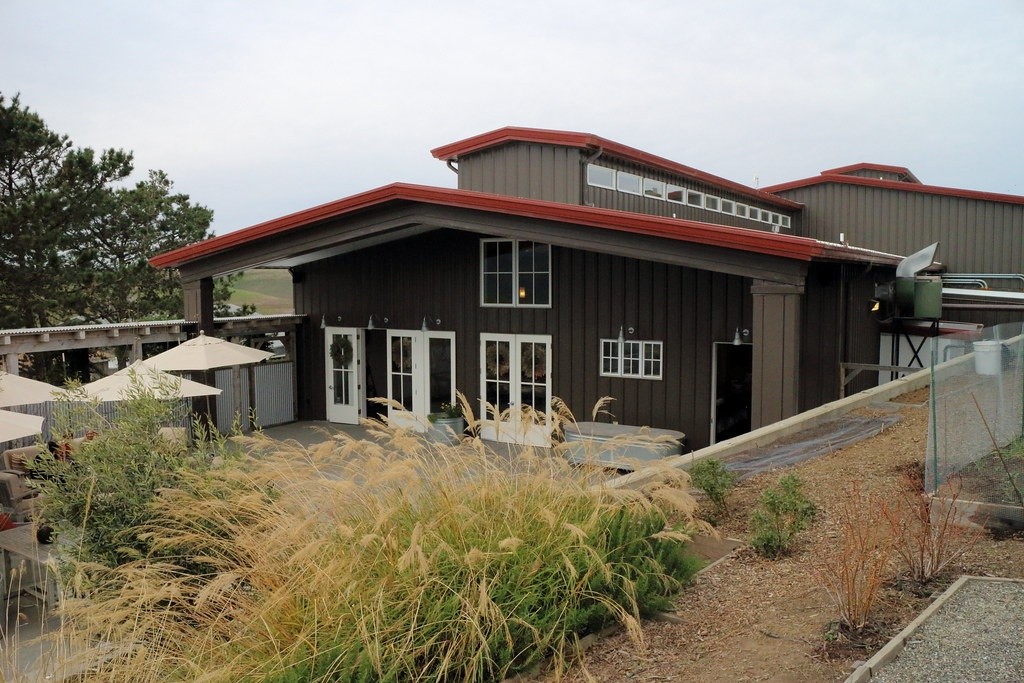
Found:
[522,346,546,378]
[392,339,412,369]
[487,344,509,375]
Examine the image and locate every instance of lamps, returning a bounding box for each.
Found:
[368,314,388,330]
[618,326,634,343]
[867,298,879,311]
[733,327,749,345]
[421,314,442,331]
[321,313,342,329]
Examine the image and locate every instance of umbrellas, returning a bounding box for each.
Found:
[73,359,224,405]
[1,409,46,447]
[144,330,276,454]
[0,371,81,409]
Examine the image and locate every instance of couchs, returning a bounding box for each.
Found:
[2,427,190,499]
[1,471,45,523]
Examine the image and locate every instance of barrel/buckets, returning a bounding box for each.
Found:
[972,341,1004,376]
[913,277,943,320]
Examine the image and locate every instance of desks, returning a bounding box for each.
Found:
[1,518,104,615]
[564,422,685,472]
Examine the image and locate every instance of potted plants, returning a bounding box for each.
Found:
[428,402,465,444]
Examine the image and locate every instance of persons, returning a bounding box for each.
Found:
[29,438,69,494]
[80,427,95,448]
[57,429,78,476]
